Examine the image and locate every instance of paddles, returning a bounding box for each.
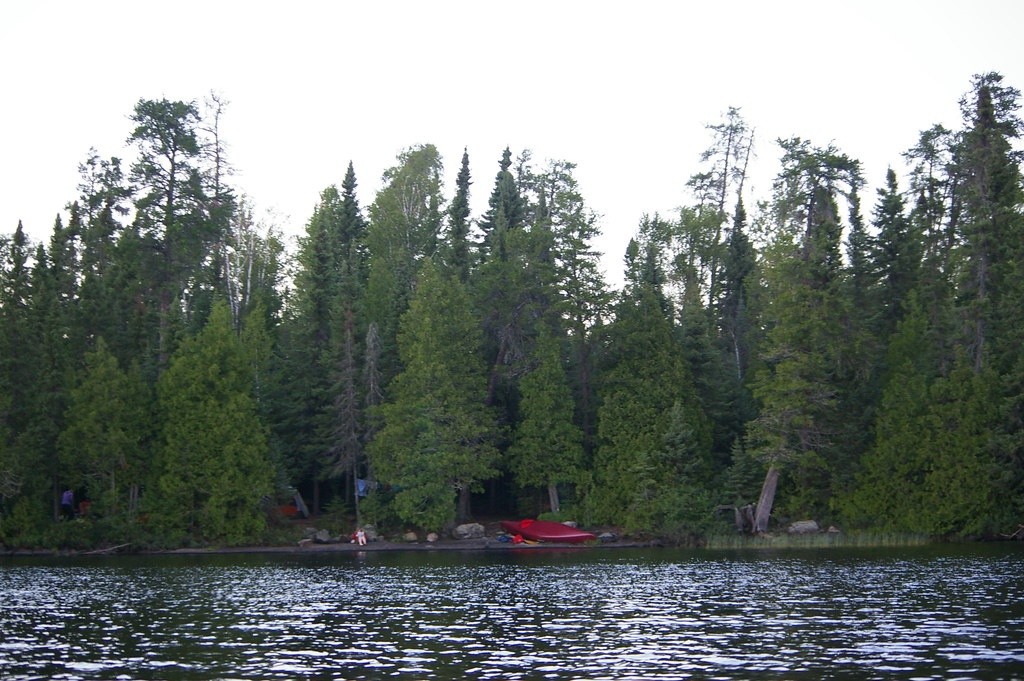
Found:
[511,535,537,544]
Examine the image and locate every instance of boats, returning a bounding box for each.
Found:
[501,520,597,544]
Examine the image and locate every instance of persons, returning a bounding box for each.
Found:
[60,489,74,522]
[80,496,91,516]
[351,527,367,546]
